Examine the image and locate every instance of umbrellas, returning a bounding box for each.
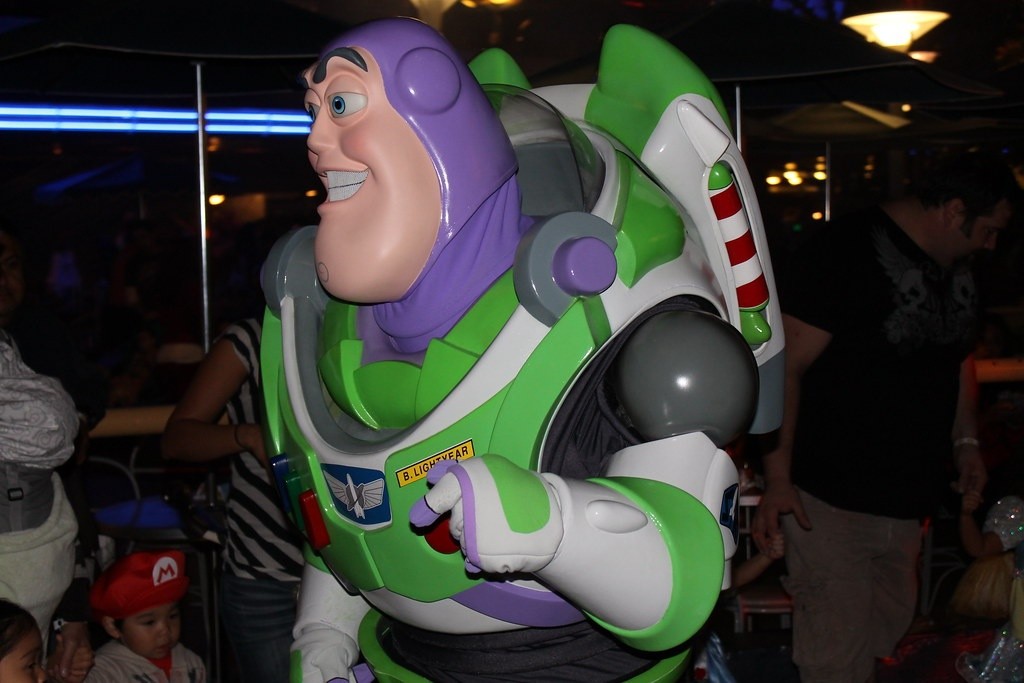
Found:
[528,0,1022,223]
[0,0,355,354]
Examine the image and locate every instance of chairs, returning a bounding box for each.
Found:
[85,433,228,683]
[723,492,796,635]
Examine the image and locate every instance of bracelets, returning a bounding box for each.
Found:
[954,436,980,448]
[233,423,252,451]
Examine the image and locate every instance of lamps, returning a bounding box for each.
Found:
[840,0,956,54]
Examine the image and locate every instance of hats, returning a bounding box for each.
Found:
[90,550,186,621]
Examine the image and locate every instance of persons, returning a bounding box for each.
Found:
[0,139,1023,683]
[262,16,760,683]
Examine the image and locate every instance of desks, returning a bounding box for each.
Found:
[87,404,232,440]
